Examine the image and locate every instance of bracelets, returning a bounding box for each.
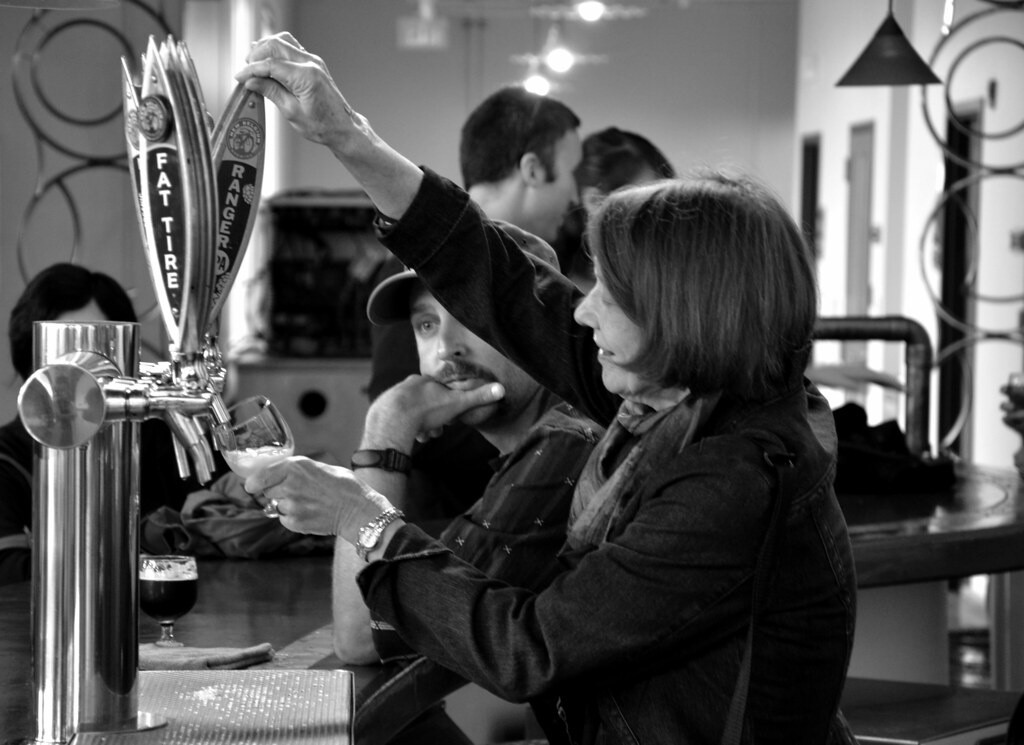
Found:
[345,444,413,476]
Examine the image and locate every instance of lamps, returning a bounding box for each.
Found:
[835,1,945,87]
[521,0,608,96]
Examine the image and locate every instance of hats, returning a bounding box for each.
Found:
[364,219,562,328]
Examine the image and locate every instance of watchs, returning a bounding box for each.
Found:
[357,507,407,565]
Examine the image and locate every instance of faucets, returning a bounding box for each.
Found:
[103,331,233,487]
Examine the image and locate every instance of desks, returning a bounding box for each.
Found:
[1,554,411,745]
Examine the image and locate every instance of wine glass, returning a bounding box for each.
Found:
[138,552,198,647]
[213,396,301,519]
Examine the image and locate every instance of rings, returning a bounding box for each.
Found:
[264,497,284,517]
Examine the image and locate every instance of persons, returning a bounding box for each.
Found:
[0,260,219,573]
[237,30,862,745]
[556,129,905,397]
[332,263,608,668]
[347,84,580,395]
